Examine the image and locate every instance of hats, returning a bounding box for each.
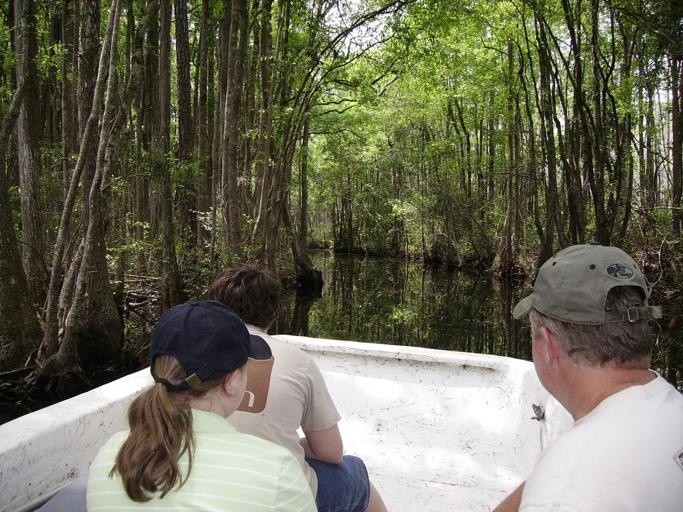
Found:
[148,297,273,392]
[510,242,664,331]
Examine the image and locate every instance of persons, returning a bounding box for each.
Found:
[493,244,683,512]
[86,298,318,512]
[201,262,387,512]
